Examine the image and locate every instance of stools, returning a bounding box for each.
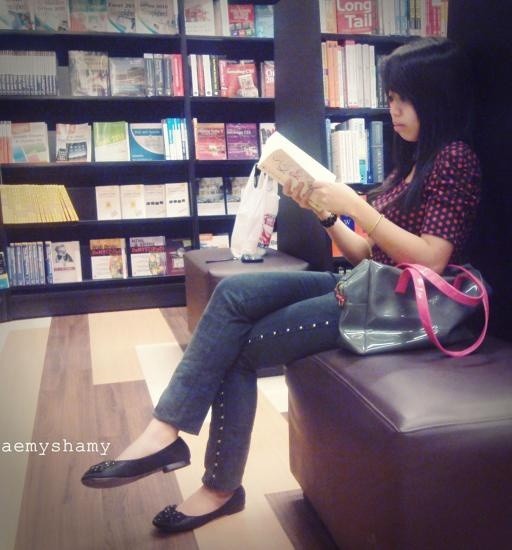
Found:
[183,243,309,335]
[279,327,511,547]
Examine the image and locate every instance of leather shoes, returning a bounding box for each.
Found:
[82,436,190,489]
[153,483,246,531]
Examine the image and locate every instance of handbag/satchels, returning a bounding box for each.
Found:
[336,258,491,358]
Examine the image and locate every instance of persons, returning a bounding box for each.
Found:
[81,32,485,533]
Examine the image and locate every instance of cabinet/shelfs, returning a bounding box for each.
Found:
[0,1,449,325]
